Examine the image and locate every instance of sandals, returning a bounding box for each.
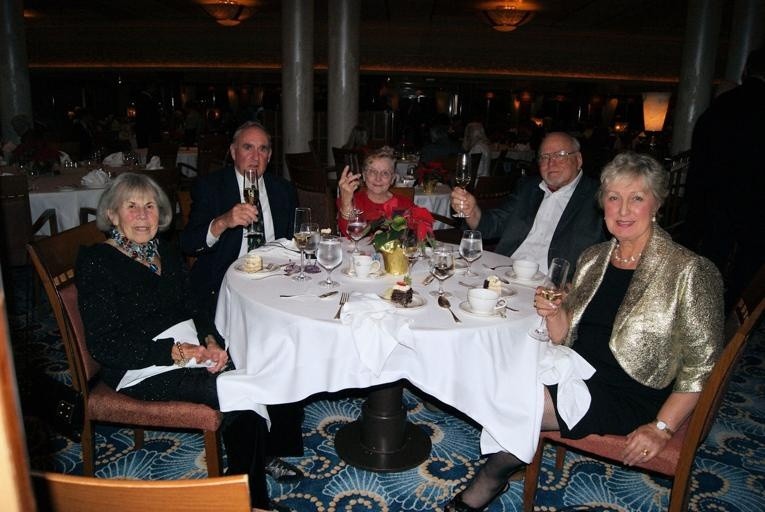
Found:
[443,478,510,512]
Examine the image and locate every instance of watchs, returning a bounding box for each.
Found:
[651,419,678,441]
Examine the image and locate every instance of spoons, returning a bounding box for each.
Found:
[436,294,462,324]
[279,290,340,302]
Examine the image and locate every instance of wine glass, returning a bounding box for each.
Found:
[528,252,570,341]
[242,169,264,239]
[451,152,472,219]
[292,207,483,300]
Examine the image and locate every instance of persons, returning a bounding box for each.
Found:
[74,173,304,512]
[452,131,603,286]
[0,91,625,204]
[431,152,728,512]
[179,120,302,323]
[336,152,412,236]
[676,50,762,338]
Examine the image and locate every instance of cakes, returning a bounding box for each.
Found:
[242,254,263,272]
[390,281,413,306]
[320,227,332,239]
[482,274,502,297]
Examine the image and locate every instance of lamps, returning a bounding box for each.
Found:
[474,0,542,31]
[641,91,671,153]
[198,0,260,26]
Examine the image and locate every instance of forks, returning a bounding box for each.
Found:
[480,263,513,270]
[333,291,350,320]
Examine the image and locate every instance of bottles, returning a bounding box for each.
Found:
[88,161,112,179]
[64,159,78,169]
[126,154,138,165]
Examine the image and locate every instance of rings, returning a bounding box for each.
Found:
[641,448,649,457]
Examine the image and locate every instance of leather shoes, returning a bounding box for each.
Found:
[266,457,304,483]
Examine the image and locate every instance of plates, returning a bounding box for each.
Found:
[504,267,545,286]
[234,262,282,275]
[460,300,507,318]
[473,283,518,298]
[379,291,427,310]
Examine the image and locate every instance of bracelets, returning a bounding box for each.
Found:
[339,207,355,220]
[175,340,185,360]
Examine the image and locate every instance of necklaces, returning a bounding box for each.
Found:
[110,229,161,274]
[612,242,642,267]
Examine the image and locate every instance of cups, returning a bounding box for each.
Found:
[512,260,539,280]
[467,288,506,312]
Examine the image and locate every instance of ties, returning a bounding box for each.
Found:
[242,184,266,253]
